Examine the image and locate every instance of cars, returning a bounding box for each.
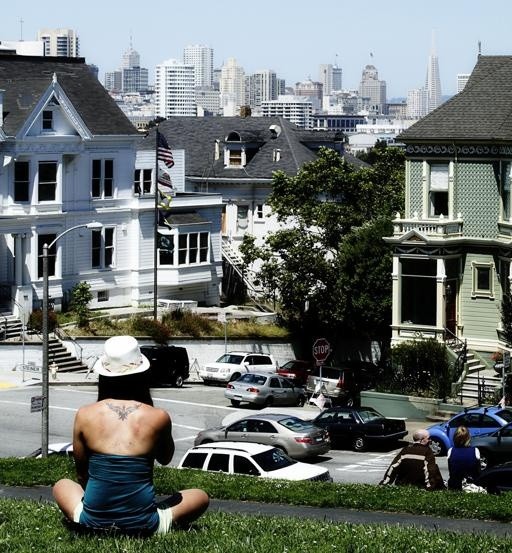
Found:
[191,411,332,460]
[424,401,511,457]
[443,418,511,471]
[17,440,76,460]
[458,461,510,496]
[221,372,306,410]
[305,402,408,454]
[271,357,314,390]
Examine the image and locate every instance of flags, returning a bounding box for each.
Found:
[157,132,174,169]
[157,166,173,189]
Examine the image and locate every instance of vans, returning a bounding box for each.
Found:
[305,364,359,408]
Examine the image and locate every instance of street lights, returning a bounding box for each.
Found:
[36,219,106,465]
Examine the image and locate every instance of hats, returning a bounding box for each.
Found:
[88,334,151,378]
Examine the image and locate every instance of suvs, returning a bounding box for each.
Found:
[177,438,334,489]
[136,342,191,389]
[198,351,279,388]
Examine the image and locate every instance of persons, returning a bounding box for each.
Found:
[379,428,447,491]
[447,425,481,491]
[52,335,209,538]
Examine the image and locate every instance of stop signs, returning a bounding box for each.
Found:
[312,338,331,362]
